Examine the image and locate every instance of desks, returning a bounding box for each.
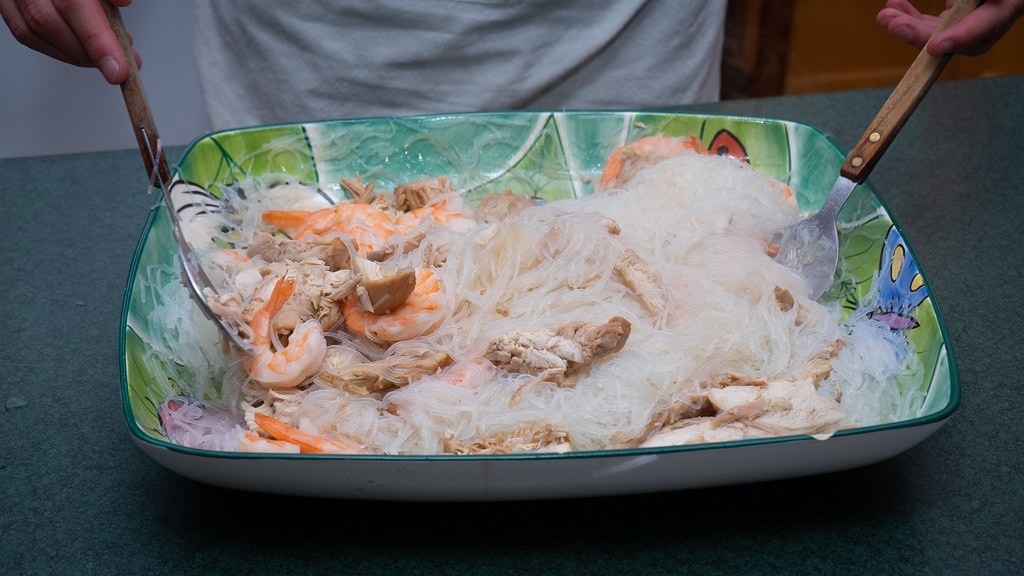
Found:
[0,72,1024,576]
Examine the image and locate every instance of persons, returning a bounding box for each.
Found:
[0,0,1023,135]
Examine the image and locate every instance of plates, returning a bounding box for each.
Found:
[118,111,960,500]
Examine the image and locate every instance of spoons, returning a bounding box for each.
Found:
[100,2,251,355]
[766,0,982,303]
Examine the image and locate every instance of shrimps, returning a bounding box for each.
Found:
[213,201,477,455]
[599,134,799,211]
